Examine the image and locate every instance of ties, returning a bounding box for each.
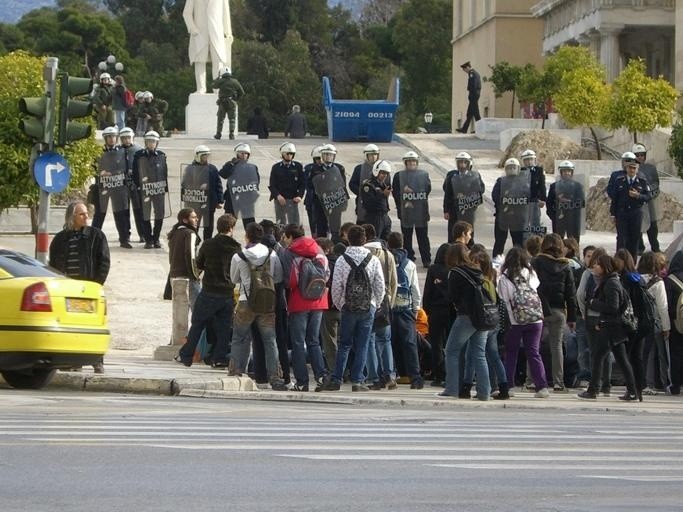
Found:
[629,178,633,186]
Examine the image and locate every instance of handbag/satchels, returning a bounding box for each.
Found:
[163,273,173,300]
[375,294,392,329]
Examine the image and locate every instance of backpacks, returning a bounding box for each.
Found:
[667,273,683,334]
[630,276,664,335]
[508,269,545,324]
[341,252,373,314]
[449,265,500,331]
[617,284,639,332]
[390,259,414,312]
[237,248,277,314]
[297,255,327,300]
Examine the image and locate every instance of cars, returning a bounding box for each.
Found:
[0,247,115,389]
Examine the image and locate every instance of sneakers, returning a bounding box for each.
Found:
[382,374,446,390]
[435,392,459,400]
[72,363,105,373]
[352,383,370,392]
[120,242,133,249]
[366,383,381,390]
[314,374,330,392]
[271,383,288,392]
[174,355,192,367]
[210,362,225,370]
[470,394,480,401]
[324,379,340,390]
[139,236,162,250]
[284,382,295,389]
[492,382,510,401]
[524,383,570,398]
[575,385,611,400]
[618,391,640,403]
[287,382,310,392]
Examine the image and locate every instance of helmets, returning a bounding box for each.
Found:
[218,66,232,77]
[279,142,296,159]
[455,152,473,169]
[311,145,322,159]
[143,130,160,146]
[119,127,135,145]
[319,143,337,160]
[503,148,538,174]
[98,65,157,105]
[372,160,391,178]
[102,126,119,141]
[233,143,251,160]
[192,145,211,163]
[363,143,380,162]
[621,142,646,168]
[558,160,575,177]
[402,151,420,166]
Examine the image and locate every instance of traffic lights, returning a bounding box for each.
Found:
[58,72,95,147]
[16,89,51,143]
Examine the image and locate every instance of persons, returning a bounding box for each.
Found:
[285,105,308,139]
[89,126,133,248]
[49,199,110,373]
[89,66,168,125]
[456,60,481,132]
[119,127,146,243]
[182,0,232,93]
[247,105,269,139]
[132,130,168,249]
[210,66,244,140]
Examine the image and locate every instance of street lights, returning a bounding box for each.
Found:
[97,55,124,83]
[423,110,434,135]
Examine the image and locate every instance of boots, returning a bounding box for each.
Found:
[458,382,473,398]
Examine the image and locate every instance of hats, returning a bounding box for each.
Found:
[460,61,471,68]
[625,158,641,168]
